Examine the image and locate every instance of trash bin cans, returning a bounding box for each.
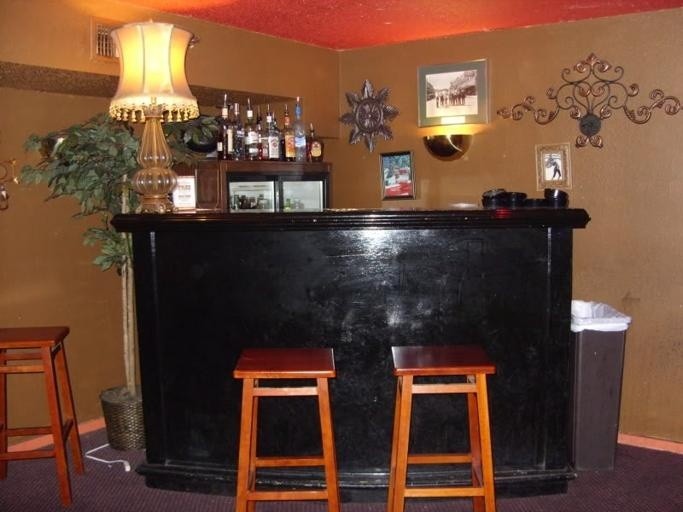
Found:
[571,300,631,472]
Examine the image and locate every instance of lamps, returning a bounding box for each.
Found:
[110,18,201,214]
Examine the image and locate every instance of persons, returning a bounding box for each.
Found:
[548,156,561,179]
[433,87,465,108]
[388,165,406,185]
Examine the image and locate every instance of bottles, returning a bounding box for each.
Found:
[215,92,324,163]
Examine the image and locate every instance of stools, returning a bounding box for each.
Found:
[387,344,498,512]
[232,346,340,511]
[0,324,86,507]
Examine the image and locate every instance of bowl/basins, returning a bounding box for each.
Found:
[481,189,568,208]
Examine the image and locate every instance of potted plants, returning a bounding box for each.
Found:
[19,109,224,451]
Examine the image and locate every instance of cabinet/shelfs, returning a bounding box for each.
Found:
[170,157,332,214]
[378,150,416,201]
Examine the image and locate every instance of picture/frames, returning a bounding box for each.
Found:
[416,59,489,127]
[534,143,573,192]
